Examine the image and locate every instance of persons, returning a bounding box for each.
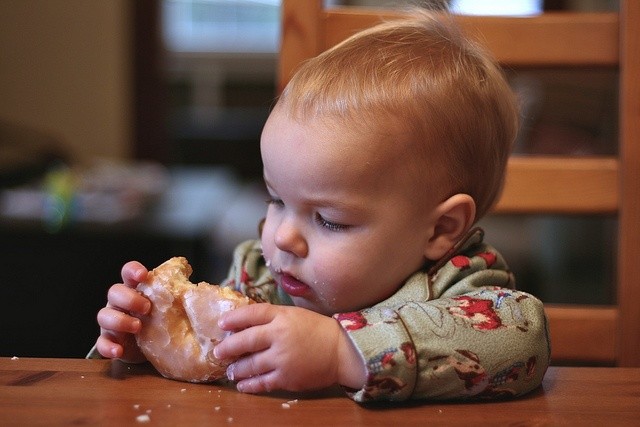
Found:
[84,5,550,409]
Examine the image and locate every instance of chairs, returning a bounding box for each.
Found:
[276,0,639,366]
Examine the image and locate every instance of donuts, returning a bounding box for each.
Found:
[131,254,256,384]
